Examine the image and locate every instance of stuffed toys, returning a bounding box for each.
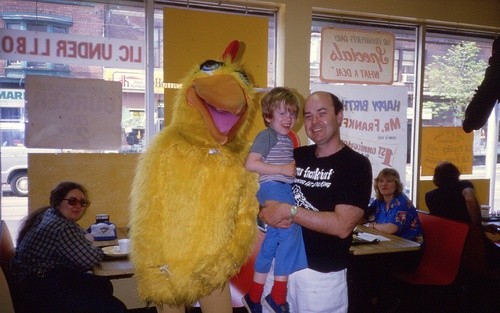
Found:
[129,40,260,313]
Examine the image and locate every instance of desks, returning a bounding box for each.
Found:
[349,224,420,255]
[92,226,135,280]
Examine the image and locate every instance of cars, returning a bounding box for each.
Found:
[1,117,165,198]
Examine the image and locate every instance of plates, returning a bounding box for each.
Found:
[101,246,128,257]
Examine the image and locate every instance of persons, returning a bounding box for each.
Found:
[10,182,127,312]
[241,87,308,313]
[425,163,500,240]
[354,168,424,313]
[259,91,372,313]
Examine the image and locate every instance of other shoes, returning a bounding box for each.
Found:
[240,293,263,313]
[263,294,290,313]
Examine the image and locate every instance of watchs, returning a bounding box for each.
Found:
[290,205,297,220]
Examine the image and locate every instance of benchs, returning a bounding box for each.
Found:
[0,220,30,313]
[400,210,469,285]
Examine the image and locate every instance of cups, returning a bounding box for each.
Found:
[96,214,109,223]
[481,205,489,216]
[118,239,130,252]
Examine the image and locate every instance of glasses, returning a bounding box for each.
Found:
[62,198,90,208]
